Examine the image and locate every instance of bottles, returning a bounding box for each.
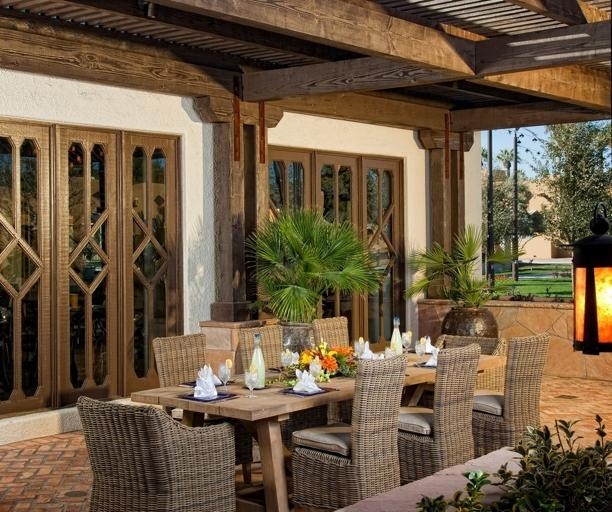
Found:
[249,333,266,389]
[391,317,402,351]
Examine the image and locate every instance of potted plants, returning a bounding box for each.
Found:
[400,220,525,338]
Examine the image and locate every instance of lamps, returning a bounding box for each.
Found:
[557,212,611,357]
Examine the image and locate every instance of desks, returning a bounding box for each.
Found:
[132,347,508,511]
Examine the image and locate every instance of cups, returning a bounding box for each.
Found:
[281,352,293,368]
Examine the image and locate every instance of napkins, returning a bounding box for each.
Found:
[293,369,322,393]
[194,365,219,400]
[202,362,221,386]
[427,348,441,367]
[422,337,434,354]
[353,337,383,360]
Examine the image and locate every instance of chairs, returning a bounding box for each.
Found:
[237,324,305,478]
[76,395,236,511]
[397,343,480,488]
[312,317,358,425]
[154,334,253,482]
[290,354,408,511]
[471,332,550,457]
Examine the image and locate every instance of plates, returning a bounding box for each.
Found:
[180,379,236,387]
[180,392,238,402]
[282,387,337,396]
[414,362,437,369]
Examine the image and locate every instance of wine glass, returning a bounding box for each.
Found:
[218,368,231,391]
[415,341,426,363]
[310,361,321,386]
[354,341,365,361]
[244,372,257,398]
[402,333,411,355]
